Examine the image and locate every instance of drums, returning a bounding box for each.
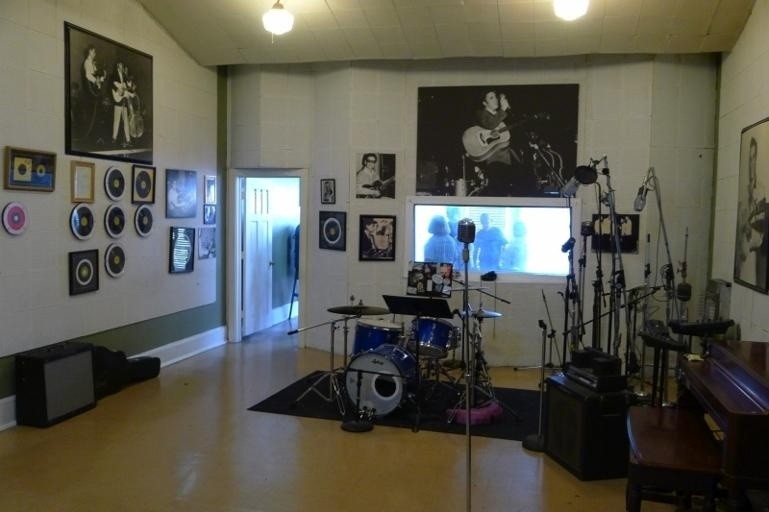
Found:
[408,316,454,360]
[354,318,407,375]
[343,342,421,421]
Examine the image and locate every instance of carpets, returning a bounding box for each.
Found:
[245,368,545,444]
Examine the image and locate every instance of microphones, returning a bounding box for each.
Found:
[677,283,691,302]
[500,94,510,109]
[458,218,475,243]
[633,186,648,211]
[559,164,598,197]
[580,221,595,236]
[561,237,576,252]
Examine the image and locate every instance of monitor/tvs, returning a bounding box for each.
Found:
[405,196,582,285]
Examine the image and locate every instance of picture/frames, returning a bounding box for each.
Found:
[733,118,769,294]
[198,225,217,259]
[65,20,154,165]
[204,205,216,224]
[105,242,126,278]
[70,204,95,240]
[1,201,27,235]
[165,169,197,218]
[359,214,396,261]
[132,165,155,203]
[318,211,346,252]
[69,249,99,296]
[5,146,56,192]
[71,159,95,202]
[134,204,153,237]
[593,213,638,254]
[105,204,127,238]
[204,175,217,204]
[169,225,195,272]
[348,147,401,204]
[105,166,126,201]
[416,84,579,198]
[320,179,335,204]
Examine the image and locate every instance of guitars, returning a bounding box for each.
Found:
[111,82,138,102]
[358,176,396,198]
[460,104,556,164]
[89,74,106,95]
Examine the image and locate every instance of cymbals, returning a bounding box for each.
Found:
[327,299,392,315]
[472,306,502,317]
[448,283,487,291]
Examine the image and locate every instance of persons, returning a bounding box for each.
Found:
[322,182,334,203]
[359,219,379,260]
[442,206,460,239]
[356,153,386,198]
[110,59,132,145]
[167,179,186,218]
[424,215,458,271]
[452,236,476,271]
[472,213,508,270]
[469,90,525,177]
[736,138,762,286]
[501,220,526,272]
[82,44,107,142]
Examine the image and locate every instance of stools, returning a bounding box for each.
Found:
[627,404,725,512]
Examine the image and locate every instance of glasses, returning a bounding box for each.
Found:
[365,159,376,164]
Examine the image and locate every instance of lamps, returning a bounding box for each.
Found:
[552,0,589,20]
[262,0,294,35]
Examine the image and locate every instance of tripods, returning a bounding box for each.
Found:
[288,316,347,419]
[511,289,566,373]
[447,320,522,427]
[433,246,492,382]
[381,315,454,433]
